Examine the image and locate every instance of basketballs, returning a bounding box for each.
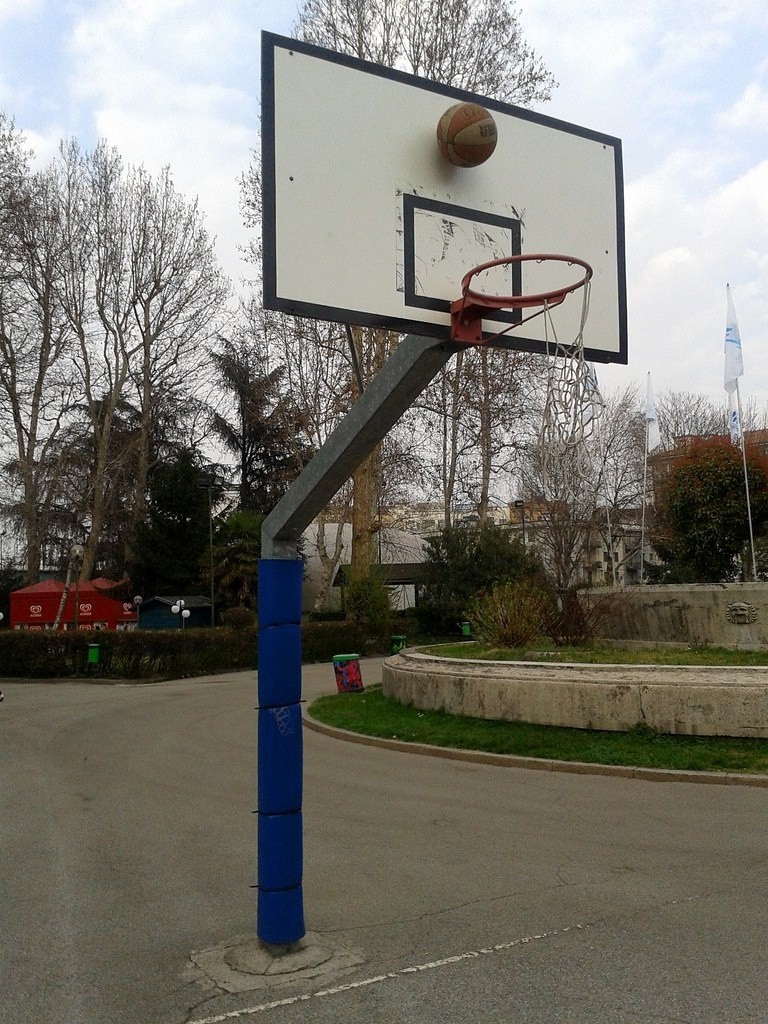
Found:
[435,103,500,171]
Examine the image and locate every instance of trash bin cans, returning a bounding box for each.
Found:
[391,635,406,656]
[461,622,471,636]
[333,654,365,694]
[88,644,100,664]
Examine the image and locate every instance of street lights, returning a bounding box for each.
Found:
[514,499,526,555]
[197,473,225,628]
